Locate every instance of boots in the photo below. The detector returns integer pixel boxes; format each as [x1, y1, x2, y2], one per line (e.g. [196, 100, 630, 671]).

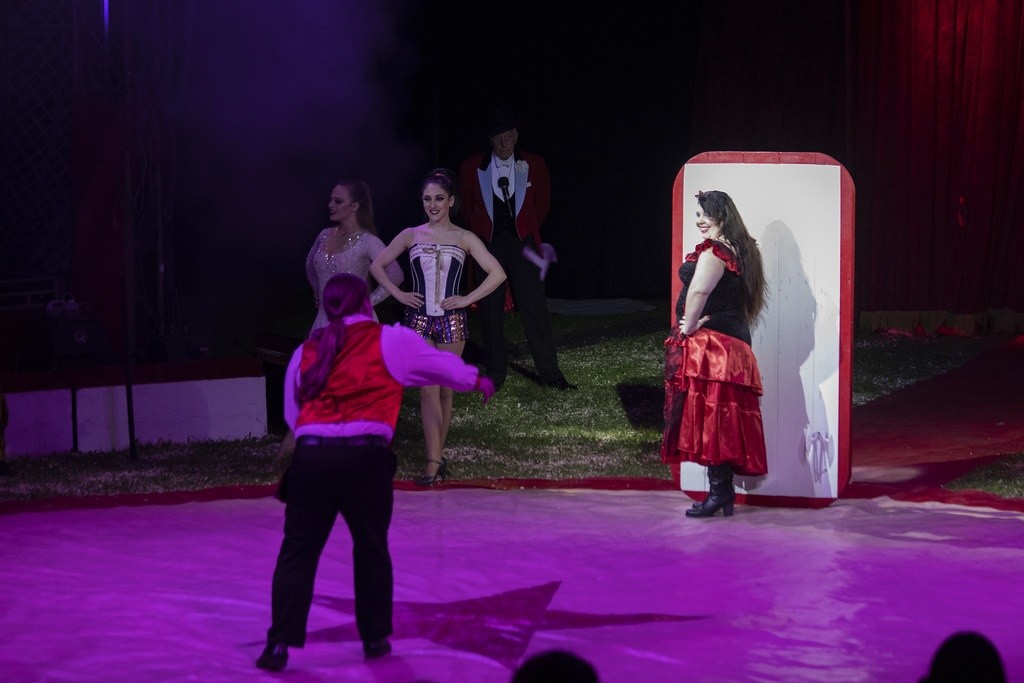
[685, 463, 734, 517]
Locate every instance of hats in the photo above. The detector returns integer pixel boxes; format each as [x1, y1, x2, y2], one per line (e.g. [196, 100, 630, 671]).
[484, 106, 520, 135]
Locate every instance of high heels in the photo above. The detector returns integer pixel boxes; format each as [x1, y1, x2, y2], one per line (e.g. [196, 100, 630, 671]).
[417, 456, 447, 485]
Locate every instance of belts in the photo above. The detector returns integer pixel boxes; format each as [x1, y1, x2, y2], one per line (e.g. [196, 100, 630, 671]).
[297, 435, 386, 447]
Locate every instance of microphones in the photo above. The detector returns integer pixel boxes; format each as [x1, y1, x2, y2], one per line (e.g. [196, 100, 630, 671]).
[497, 176, 513, 216]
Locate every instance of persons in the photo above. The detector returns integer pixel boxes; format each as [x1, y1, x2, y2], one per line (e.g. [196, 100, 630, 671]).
[255, 274, 497, 670]
[661, 191, 767, 517]
[369, 168, 508, 485]
[306, 181, 405, 337]
[457, 114, 579, 391]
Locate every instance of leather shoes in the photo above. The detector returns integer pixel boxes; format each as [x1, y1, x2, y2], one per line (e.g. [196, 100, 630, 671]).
[256, 642, 287, 671]
[364, 639, 391, 658]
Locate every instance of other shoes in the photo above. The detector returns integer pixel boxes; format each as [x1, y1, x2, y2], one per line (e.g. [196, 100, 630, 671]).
[493, 383, 502, 392]
[541, 377, 577, 391]
